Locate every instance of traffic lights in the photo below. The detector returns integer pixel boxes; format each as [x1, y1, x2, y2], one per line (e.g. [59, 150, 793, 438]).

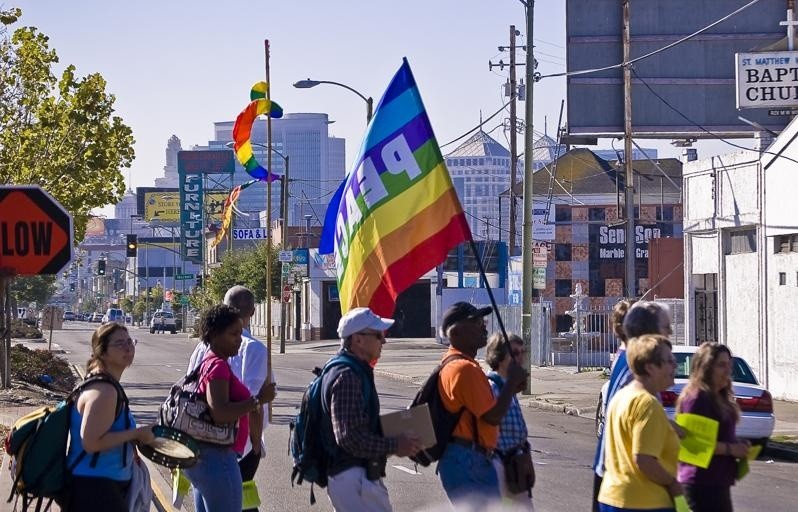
[126, 233, 137, 257]
[98, 261, 105, 275]
[70, 283, 74, 291]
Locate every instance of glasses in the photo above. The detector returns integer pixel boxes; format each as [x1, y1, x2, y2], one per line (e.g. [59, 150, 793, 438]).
[108, 339, 137, 348]
[357, 331, 382, 339]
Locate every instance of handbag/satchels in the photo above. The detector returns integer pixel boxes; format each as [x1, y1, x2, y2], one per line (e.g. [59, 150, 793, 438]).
[159, 355, 240, 446]
[500, 441, 536, 493]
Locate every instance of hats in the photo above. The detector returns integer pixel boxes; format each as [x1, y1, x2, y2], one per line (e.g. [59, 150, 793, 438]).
[442, 302, 493, 338]
[336, 307, 396, 338]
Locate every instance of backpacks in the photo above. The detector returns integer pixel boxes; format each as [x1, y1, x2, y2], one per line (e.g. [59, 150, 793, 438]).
[287, 355, 372, 485]
[407, 354, 468, 466]
[5, 374, 126, 496]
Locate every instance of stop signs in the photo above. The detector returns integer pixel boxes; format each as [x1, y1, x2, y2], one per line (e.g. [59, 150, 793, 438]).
[282, 286, 291, 303]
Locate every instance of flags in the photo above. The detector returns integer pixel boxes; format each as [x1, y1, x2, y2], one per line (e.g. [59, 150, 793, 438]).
[318, 61, 471, 371]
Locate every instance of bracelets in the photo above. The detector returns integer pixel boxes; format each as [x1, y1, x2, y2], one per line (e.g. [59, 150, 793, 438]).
[252, 395, 261, 412]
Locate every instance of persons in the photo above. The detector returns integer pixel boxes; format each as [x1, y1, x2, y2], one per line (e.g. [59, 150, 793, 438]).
[59, 321, 161, 512]
[589, 297, 750, 510]
[182, 303, 276, 511]
[322, 307, 426, 511]
[435, 301, 530, 511]
[187, 284, 271, 511]
[484, 331, 537, 511]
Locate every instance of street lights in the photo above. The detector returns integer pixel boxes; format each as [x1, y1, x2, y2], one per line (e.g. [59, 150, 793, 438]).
[160, 195, 218, 276]
[290, 77, 374, 135]
[226, 139, 288, 354]
[570, 282, 588, 372]
[142, 224, 175, 295]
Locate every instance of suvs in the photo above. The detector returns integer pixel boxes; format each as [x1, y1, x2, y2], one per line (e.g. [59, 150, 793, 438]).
[149, 311, 177, 335]
[102, 309, 124, 325]
[63, 311, 74, 322]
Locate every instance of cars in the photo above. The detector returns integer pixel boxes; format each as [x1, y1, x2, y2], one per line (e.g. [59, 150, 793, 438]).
[595, 343, 780, 457]
[75, 311, 103, 323]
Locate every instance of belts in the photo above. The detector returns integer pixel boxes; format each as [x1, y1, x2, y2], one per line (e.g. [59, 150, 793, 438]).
[450, 436, 494, 460]
